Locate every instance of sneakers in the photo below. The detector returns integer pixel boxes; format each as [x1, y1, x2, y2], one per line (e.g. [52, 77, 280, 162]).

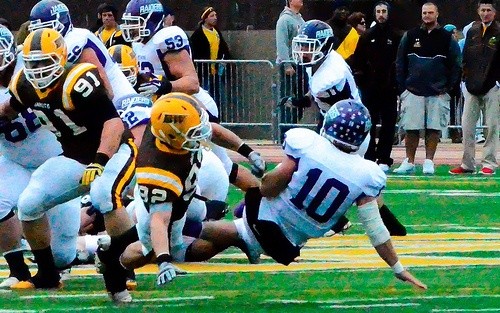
[95, 243, 108, 273]
[393, 156, 436, 174]
[475, 133, 485, 142]
[118, 253, 137, 289]
[378, 163, 389, 171]
[233, 216, 261, 264]
[234, 198, 246, 218]
[103, 261, 132, 303]
[323, 215, 351, 236]
[449, 165, 497, 174]
[11, 274, 63, 291]
[0, 272, 32, 288]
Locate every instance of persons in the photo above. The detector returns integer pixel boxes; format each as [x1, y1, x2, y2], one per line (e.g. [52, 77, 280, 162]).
[0, 0, 500, 304]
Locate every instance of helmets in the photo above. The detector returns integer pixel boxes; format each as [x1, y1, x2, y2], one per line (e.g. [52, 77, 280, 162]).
[443, 23, 457, 32]
[0, 0, 70, 73]
[297, 18, 334, 58]
[319, 98, 371, 150]
[150, 91, 208, 151]
[125, 0, 165, 36]
[113, 93, 153, 128]
[107, 44, 139, 76]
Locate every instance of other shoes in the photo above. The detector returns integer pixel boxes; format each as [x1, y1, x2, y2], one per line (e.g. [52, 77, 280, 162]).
[451, 133, 462, 143]
[440, 137, 452, 143]
[401, 138, 425, 146]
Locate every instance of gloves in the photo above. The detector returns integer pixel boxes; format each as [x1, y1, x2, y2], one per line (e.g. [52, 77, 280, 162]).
[156, 261, 187, 284]
[276, 95, 298, 108]
[79, 162, 105, 184]
[138, 71, 172, 96]
[249, 150, 266, 176]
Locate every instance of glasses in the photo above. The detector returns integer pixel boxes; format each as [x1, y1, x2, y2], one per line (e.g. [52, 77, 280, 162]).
[358, 20, 366, 25]
